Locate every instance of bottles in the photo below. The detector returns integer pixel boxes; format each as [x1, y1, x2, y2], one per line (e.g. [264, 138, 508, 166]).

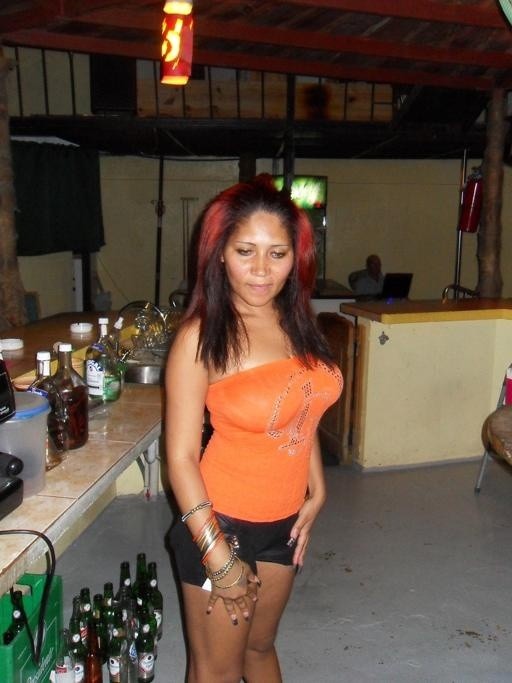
[5, 552, 165, 683]
[25, 312, 128, 471]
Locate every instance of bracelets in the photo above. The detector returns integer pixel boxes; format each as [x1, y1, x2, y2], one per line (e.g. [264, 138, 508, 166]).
[182, 501, 243, 588]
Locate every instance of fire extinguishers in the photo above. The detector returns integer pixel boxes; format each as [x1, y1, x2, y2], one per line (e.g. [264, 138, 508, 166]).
[459, 161, 484, 233]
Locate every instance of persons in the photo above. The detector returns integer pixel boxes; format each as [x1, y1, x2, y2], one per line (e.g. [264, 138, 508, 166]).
[354, 254, 385, 295]
[164, 175, 344, 683]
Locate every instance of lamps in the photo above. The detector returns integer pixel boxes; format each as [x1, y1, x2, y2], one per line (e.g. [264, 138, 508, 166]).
[158, 13, 195, 86]
[161, 0, 193, 15]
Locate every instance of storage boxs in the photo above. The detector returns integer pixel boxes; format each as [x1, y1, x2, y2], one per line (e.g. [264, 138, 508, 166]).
[1, 573, 67, 683]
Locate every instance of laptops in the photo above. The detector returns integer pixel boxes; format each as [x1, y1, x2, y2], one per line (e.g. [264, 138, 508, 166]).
[380, 274, 412, 298]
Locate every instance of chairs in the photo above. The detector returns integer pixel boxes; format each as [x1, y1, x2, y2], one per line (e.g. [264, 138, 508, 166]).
[473, 363, 512, 494]
